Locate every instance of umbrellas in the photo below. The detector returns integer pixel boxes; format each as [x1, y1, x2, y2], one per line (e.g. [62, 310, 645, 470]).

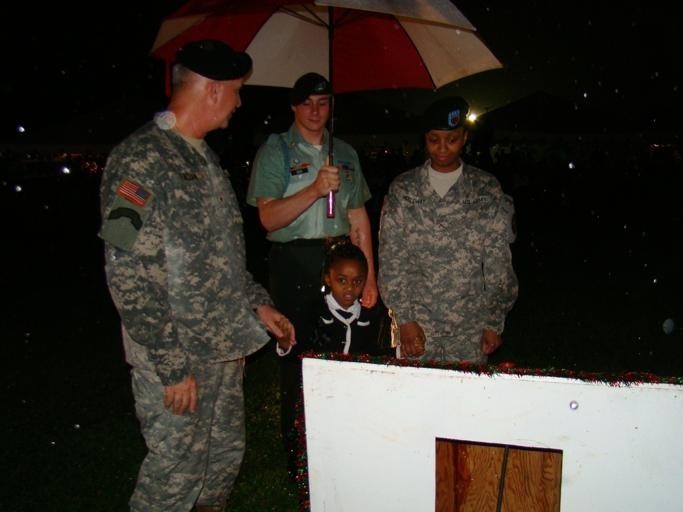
[147, 0, 505, 97]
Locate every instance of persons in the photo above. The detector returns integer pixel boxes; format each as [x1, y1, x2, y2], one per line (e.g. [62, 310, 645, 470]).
[275, 236, 426, 467]
[98, 36, 298, 511]
[374, 93, 522, 364]
[245, 71, 379, 449]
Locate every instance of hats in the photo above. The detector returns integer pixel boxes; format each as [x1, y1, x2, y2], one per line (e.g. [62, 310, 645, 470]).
[174, 39, 252, 81]
[290, 73, 333, 105]
[412, 97, 469, 134]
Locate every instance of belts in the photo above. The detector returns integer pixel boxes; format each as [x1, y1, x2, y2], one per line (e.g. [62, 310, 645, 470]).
[271, 234, 348, 249]
